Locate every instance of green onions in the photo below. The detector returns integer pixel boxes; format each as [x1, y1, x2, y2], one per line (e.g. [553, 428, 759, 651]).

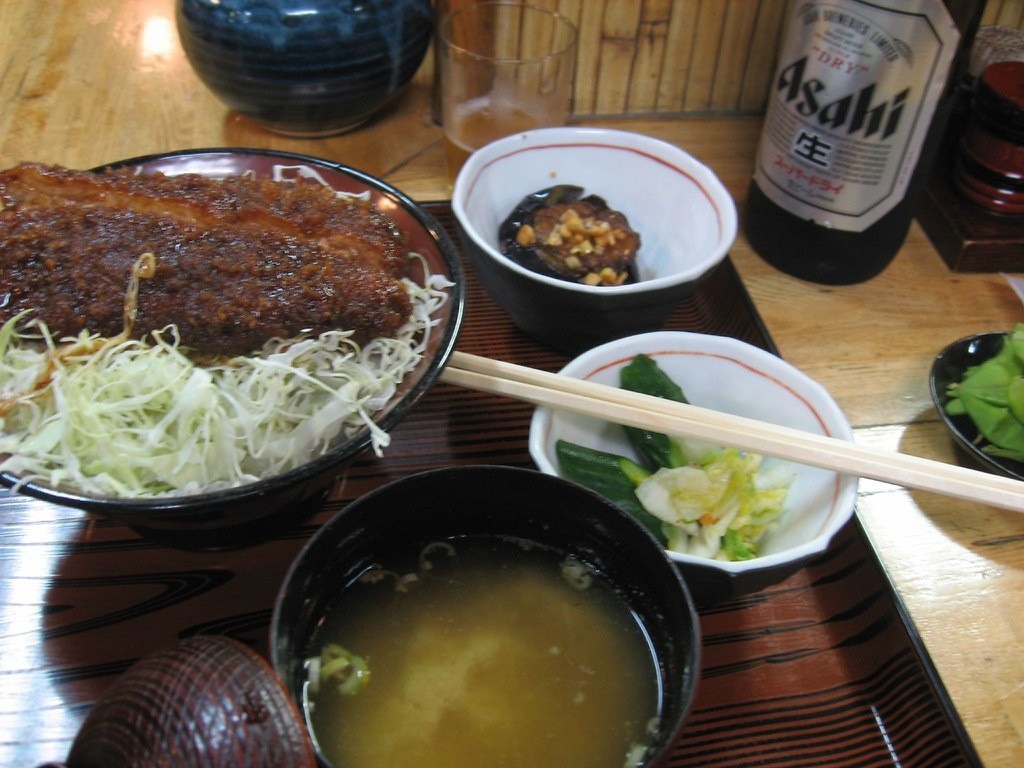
[296, 542, 663, 768]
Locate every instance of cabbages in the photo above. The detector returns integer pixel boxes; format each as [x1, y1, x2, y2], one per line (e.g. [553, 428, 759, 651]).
[635, 438, 793, 566]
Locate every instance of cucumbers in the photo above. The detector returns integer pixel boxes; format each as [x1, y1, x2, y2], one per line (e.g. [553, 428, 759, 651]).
[554, 353, 707, 545]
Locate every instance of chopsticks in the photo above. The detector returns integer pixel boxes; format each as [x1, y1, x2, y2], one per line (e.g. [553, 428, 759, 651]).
[435, 345, 1024, 528]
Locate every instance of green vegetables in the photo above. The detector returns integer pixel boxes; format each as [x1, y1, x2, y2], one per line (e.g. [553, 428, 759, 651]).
[945, 318, 1023, 469]
[0, 164, 458, 498]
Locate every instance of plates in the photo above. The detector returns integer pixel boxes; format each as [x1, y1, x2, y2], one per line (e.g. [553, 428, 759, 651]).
[430, 2, 578, 149]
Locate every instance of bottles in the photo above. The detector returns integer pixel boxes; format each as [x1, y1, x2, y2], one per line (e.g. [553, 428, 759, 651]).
[740, 0, 993, 286]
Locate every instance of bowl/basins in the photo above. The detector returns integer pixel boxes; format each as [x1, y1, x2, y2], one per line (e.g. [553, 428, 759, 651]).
[929, 329, 1024, 482]
[527, 330, 856, 598]
[0, 144, 469, 551]
[449, 127, 738, 351]
[268, 463, 700, 768]
